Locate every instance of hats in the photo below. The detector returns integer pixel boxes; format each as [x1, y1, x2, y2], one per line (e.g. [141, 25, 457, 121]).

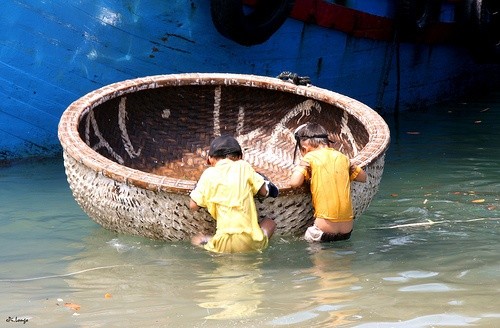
[206, 134, 242, 165]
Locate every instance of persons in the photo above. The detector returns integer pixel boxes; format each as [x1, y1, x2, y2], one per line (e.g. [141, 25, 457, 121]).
[289, 123, 367, 244]
[190, 134, 277, 257]
